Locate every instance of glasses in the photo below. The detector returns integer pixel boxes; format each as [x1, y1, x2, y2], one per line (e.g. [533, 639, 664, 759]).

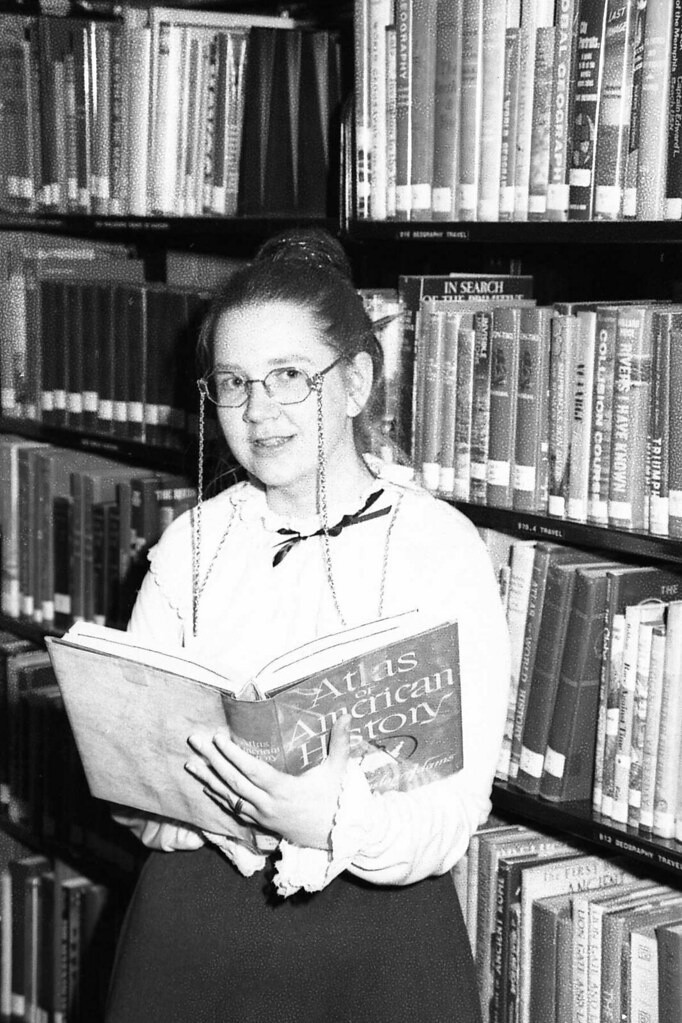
[199, 344, 367, 408]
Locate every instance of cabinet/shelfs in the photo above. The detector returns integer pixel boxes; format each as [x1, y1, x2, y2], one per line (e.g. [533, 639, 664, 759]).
[0, 0, 682, 895]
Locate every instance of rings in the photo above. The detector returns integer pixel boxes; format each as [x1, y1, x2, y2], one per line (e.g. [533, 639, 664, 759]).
[233, 798, 245, 814]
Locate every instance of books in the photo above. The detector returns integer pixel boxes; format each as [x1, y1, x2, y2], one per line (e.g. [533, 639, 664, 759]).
[2, 1, 682, 1021]
[43, 607, 474, 859]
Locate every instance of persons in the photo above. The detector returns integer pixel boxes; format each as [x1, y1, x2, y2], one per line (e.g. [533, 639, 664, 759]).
[102, 235, 514, 1023]
